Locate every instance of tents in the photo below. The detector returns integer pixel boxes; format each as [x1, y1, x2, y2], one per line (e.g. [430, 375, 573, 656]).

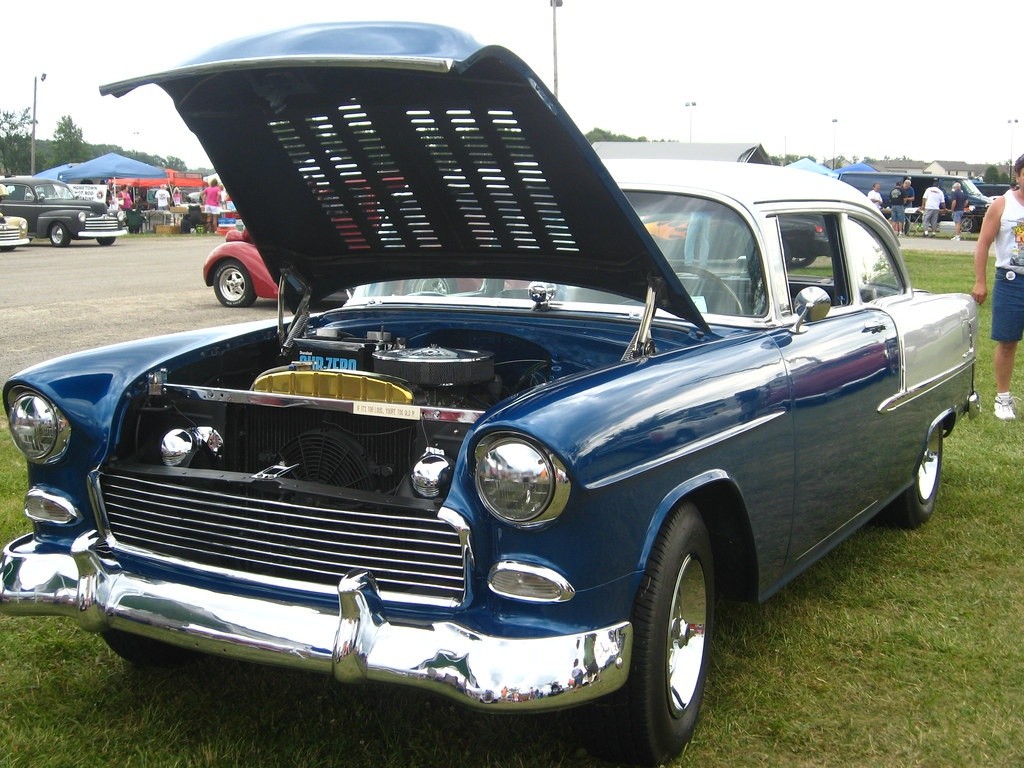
[108, 169, 209, 204]
[57, 152, 177, 207]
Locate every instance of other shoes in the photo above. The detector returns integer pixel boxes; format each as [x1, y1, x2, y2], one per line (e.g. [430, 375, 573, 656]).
[214, 230, 217, 234]
[929, 234, 936, 238]
[921, 234, 928, 237]
[207, 230, 210, 234]
[951, 236, 960, 241]
[898, 233, 905, 236]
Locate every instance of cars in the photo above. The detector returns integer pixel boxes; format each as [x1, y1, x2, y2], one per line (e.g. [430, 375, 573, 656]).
[0, 24, 979, 768]
[203, 178, 408, 307]
[0, 213, 30, 250]
[0, 175, 127, 247]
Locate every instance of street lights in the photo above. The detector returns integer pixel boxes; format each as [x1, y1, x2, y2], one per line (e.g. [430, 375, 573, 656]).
[550, 0, 563, 100]
[1008, 118, 1018, 181]
[832, 119, 837, 169]
[684, 102, 695, 143]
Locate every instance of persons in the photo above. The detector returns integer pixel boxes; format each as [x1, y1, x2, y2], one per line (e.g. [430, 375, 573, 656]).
[971, 153, 1024, 420]
[951, 182, 968, 241]
[200, 178, 232, 234]
[100, 180, 182, 225]
[868, 180, 948, 238]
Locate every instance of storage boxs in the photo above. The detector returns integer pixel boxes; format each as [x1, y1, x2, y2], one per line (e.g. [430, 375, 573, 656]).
[156, 225, 180, 234]
[220, 211, 242, 218]
[217, 228, 237, 236]
[218, 218, 236, 227]
[170, 207, 187, 213]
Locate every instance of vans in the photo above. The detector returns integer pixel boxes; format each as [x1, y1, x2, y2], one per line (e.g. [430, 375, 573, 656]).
[841, 171, 993, 233]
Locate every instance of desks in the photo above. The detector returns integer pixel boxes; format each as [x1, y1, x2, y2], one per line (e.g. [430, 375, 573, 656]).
[157, 211, 211, 226]
[880, 208, 952, 237]
[120, 210, 157, 234]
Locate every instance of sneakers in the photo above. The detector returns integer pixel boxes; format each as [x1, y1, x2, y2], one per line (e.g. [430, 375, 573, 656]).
[993, 395, 1023, 422]
